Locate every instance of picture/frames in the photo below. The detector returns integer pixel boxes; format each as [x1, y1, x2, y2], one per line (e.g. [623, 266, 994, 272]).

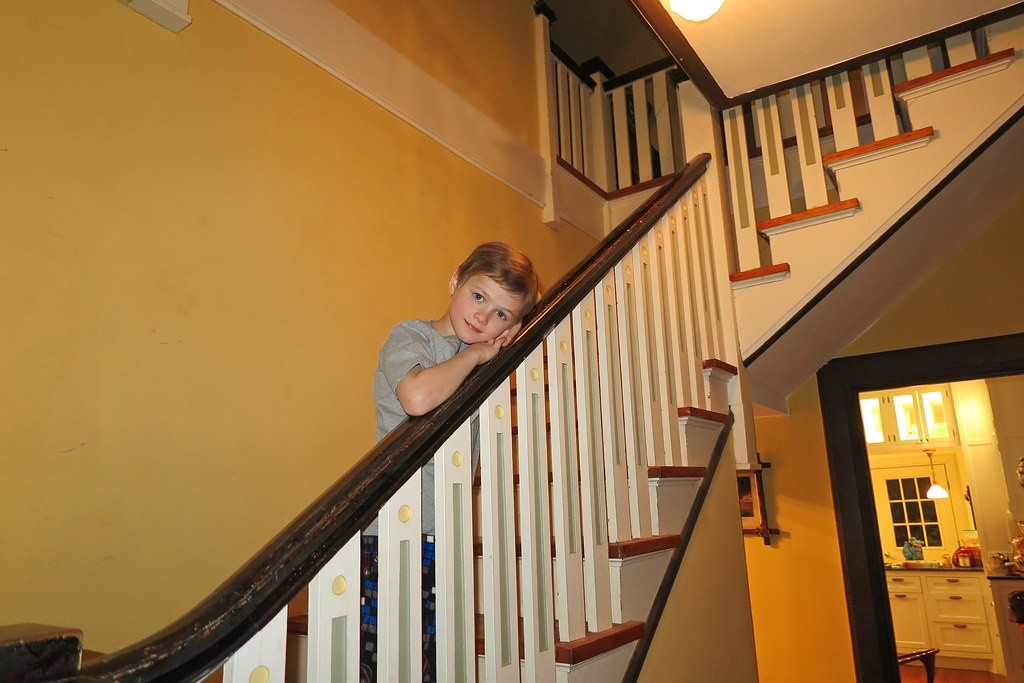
[735, 459, 778, 545]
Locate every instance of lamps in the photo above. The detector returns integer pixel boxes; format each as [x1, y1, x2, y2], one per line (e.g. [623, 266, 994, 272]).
[922, 449, 949, 499]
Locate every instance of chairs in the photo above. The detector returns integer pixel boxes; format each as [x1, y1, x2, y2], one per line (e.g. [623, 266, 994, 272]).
[897, 648, 939, 683]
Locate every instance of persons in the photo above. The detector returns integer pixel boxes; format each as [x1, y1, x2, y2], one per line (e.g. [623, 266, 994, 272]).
[360, 241, 539, 683]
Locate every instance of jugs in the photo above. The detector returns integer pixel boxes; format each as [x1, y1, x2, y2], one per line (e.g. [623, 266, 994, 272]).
[940, 553, 952, 569]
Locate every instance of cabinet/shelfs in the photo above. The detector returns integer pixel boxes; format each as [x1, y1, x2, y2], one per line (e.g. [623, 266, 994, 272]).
[858, 383, 961, 454]
[886, 571, 1004, 675]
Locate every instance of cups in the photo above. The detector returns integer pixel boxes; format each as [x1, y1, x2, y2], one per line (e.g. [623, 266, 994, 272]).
[1004, 562, 1017, 575]
